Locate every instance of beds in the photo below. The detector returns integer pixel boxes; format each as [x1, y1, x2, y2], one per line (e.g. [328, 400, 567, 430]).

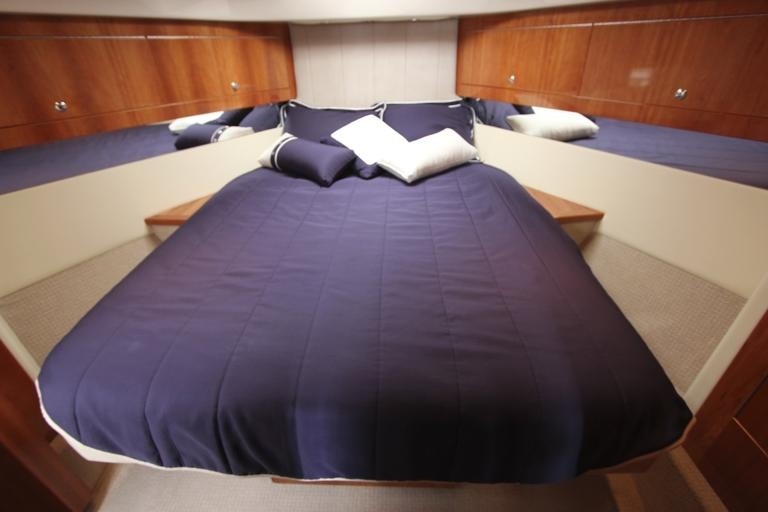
[33, 99, 702, 490]
[0, 123, 181, 198]
[564, 114, 768, 193]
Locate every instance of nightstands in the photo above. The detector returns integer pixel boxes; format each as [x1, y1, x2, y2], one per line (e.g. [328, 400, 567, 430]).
[520, 187, 605, 252]
[144, 193, 215, 243]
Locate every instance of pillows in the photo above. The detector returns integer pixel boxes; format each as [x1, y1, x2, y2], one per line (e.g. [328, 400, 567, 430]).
[168, 103, 281, 152]
[463, 96, 600, 142]
[258, 100, 485, 187]
